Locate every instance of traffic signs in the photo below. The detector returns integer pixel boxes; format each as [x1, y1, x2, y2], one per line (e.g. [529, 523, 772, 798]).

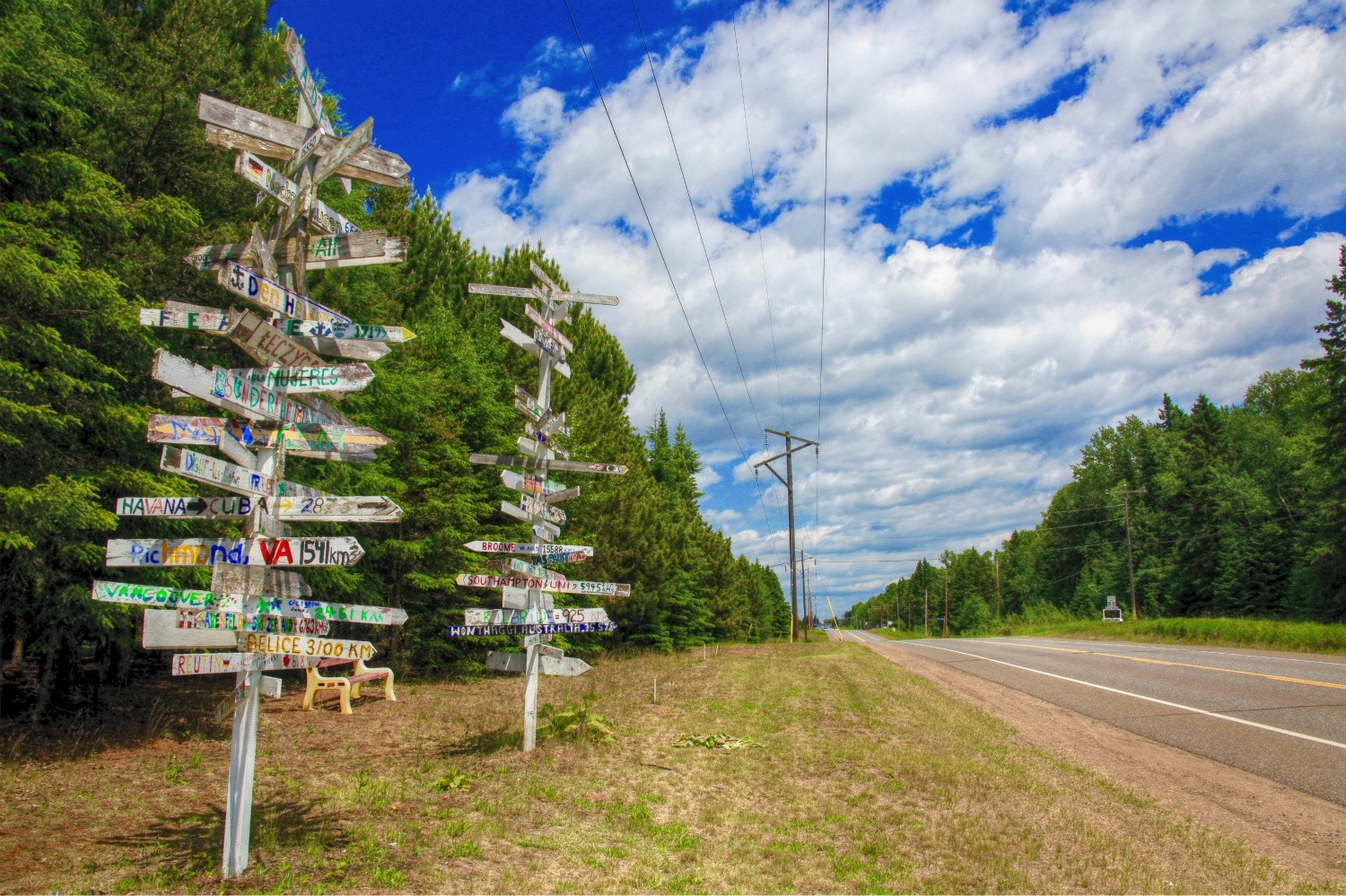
[92, 20, 421, 678]
[467, 259, 618, 380]
[449, 384, 633, 676]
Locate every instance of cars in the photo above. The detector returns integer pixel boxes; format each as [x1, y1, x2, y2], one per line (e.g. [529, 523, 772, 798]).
[823, 627, 829, 631]
[881, 624, 886, 628]
[812, 624, 819, 630]
